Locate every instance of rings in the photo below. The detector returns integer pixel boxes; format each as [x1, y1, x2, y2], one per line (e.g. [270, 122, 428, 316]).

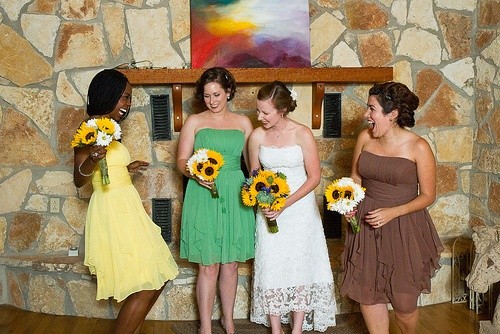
[93, 152, 99, 157]
[377, 222, 379, 225]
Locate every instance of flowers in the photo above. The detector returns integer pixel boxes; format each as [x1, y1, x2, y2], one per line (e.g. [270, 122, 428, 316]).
[325, 177, 366, 233]
[243, 168, 291, 233]
[71, 118, 121, 185]
[187, 148, 225, 200]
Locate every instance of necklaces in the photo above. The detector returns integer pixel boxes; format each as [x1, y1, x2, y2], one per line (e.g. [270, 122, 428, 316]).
[273, 120, 287, 138]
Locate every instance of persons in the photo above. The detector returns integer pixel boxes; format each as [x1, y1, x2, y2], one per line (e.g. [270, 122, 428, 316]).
[248, 81, 341, 334]
[176, 66, 254, 334]
[341, 81, 446, 334]
[73, 68, 180, 334]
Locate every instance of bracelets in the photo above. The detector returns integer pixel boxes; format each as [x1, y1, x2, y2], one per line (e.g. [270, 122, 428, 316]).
[78, 163, 95, 177]
